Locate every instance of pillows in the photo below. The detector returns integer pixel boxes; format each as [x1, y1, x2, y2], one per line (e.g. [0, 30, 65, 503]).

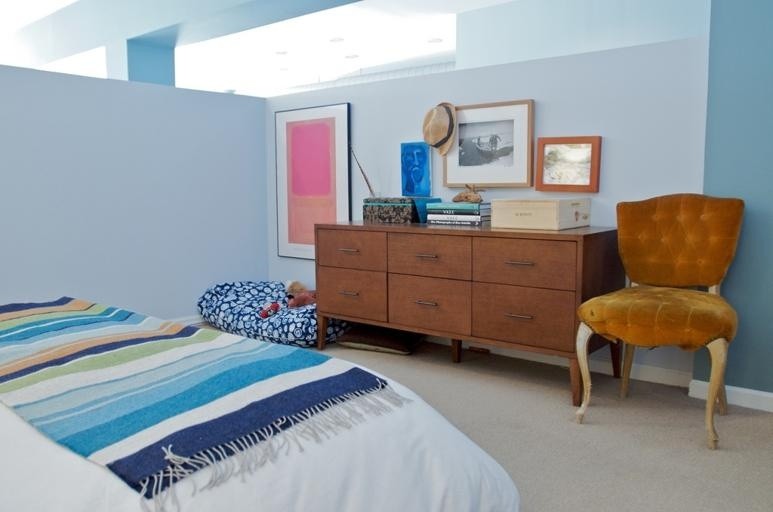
[334, 320, 427, 356]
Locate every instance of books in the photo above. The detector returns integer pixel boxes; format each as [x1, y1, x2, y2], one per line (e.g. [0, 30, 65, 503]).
[424, 200, 491, 227]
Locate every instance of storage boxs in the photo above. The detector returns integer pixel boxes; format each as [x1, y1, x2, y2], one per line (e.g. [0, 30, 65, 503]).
[360, 197, 418, 224]
[489, 199, 592, 231]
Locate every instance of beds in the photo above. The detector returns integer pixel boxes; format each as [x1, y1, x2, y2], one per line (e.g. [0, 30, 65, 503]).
[0, 296, 521, 511]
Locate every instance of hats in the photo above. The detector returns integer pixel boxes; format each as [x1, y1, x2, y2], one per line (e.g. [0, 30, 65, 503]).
[422, 103, 457, 156]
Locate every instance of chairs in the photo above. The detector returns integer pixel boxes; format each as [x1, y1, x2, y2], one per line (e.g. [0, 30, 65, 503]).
[575, 195, 747, 447]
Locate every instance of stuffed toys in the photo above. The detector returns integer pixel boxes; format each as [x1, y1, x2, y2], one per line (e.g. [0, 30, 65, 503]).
[258, 279, 317, 320]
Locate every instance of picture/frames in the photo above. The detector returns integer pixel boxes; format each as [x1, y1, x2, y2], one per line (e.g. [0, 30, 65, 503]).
[438, 103, 601, 193]
[271, 98, 355, 260]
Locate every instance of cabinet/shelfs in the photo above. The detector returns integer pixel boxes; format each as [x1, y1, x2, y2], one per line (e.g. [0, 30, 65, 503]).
[314, 219, 623, 405]
